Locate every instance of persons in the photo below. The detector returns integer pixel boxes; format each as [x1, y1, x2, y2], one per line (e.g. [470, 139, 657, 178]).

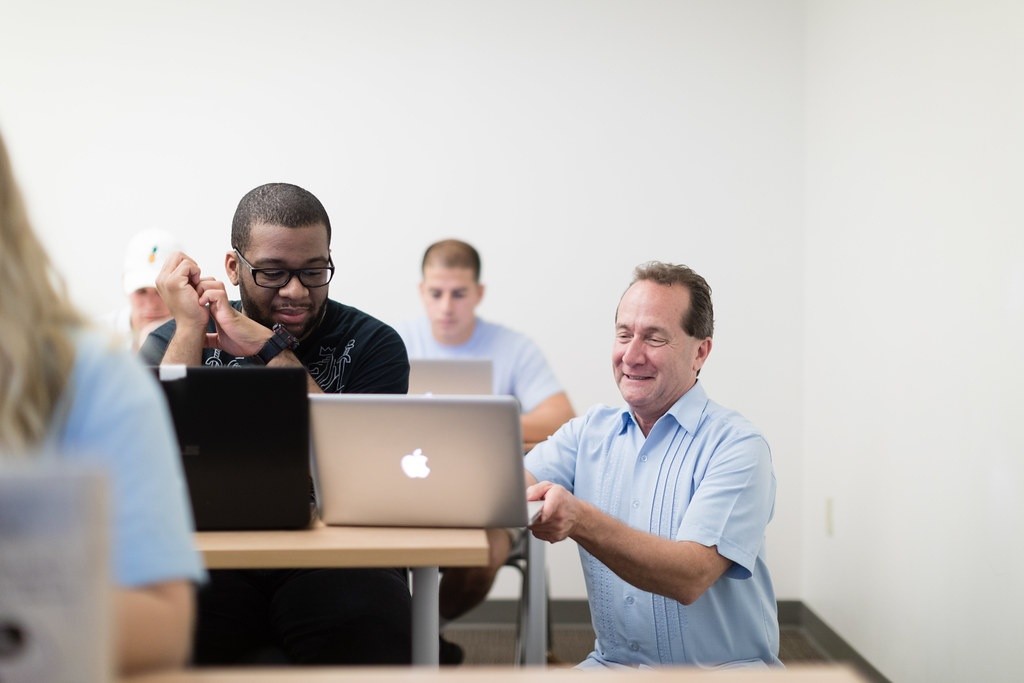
[395, 240, 577, 667]
[104, 230, 175, 353]
[135, 182, 465, 668]
[520, 257, 787, 677]
[0, 126, 209, 675]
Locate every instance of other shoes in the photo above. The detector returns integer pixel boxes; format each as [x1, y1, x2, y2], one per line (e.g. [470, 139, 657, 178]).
[439, 634, 465, 666]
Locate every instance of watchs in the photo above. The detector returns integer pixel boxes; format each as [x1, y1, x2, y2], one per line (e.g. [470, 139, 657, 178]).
[244, 322, 299, 365]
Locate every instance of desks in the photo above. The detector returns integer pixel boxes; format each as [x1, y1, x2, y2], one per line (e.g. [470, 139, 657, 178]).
[182, 502, 551, 665]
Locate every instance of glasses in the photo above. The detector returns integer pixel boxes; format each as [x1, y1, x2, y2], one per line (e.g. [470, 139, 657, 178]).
[233, 247, 335, 289]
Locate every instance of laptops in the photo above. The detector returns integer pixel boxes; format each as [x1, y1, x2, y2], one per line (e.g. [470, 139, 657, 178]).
[406, 358, 493, 396]
[308, 393, 545, 527]
[147, 365, 318, 532]
